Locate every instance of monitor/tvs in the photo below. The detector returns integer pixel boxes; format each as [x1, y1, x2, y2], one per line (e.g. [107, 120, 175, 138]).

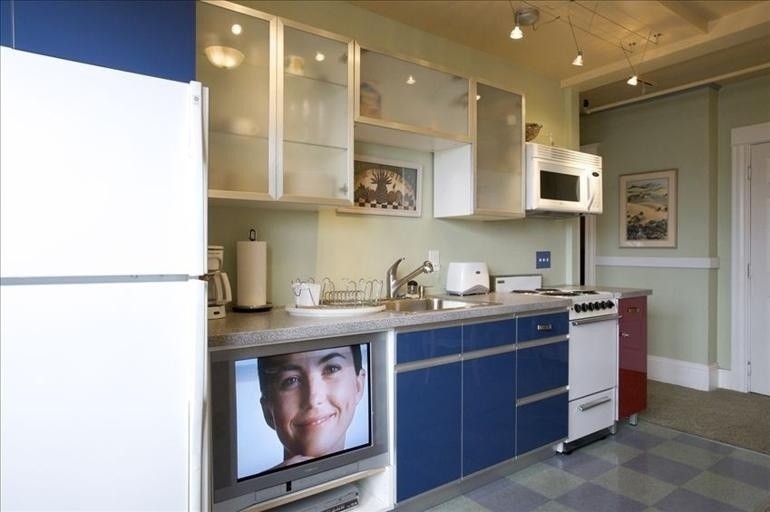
[208, 332, 392, 511]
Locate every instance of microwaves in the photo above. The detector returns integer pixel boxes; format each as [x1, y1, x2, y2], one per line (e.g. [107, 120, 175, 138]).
[526, 141, 603, 215]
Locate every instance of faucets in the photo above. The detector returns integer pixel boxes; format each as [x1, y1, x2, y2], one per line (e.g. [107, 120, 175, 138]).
[385, 257, 434, 299]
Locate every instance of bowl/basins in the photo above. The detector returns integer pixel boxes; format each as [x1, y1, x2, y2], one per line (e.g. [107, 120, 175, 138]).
[291, 282, 321, 305]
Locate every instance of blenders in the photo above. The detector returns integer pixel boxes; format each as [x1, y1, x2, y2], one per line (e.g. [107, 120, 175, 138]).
[207, 245, 233, 320]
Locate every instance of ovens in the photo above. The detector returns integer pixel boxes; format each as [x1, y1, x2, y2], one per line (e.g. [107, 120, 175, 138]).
[558, 295, 621, 455]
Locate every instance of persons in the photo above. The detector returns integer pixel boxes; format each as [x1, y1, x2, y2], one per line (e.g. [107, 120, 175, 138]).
[258, 343, 366, 472]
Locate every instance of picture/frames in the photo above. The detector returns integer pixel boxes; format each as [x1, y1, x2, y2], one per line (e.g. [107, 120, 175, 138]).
[617, 165, 680, 253]
[337, 153, 426, 219]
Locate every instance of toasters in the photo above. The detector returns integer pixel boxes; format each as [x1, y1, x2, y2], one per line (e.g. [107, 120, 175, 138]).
[445, 262, 490, 296]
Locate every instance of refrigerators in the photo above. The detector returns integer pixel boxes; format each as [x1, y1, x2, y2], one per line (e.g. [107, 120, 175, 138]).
[0, 45, 214, 512]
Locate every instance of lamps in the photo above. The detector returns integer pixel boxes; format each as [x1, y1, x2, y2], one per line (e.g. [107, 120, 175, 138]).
[313, 49, 326, 62]
[505, 2, 660, 88]
[202, 45, 245, 71]
[407, 74, 415, 84]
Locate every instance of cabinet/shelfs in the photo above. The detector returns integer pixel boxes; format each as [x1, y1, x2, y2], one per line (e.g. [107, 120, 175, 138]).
[517, 307, 569, 461]
[195, 0, 357, 208]
[395, 313, 518, 496]
[357, 42, 472, 153]
[618, 293, 652, 425]
[432, 79, 529, 223]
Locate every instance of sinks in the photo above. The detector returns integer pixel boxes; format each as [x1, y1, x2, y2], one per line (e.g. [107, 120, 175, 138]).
[373, 294, 504, 317]
